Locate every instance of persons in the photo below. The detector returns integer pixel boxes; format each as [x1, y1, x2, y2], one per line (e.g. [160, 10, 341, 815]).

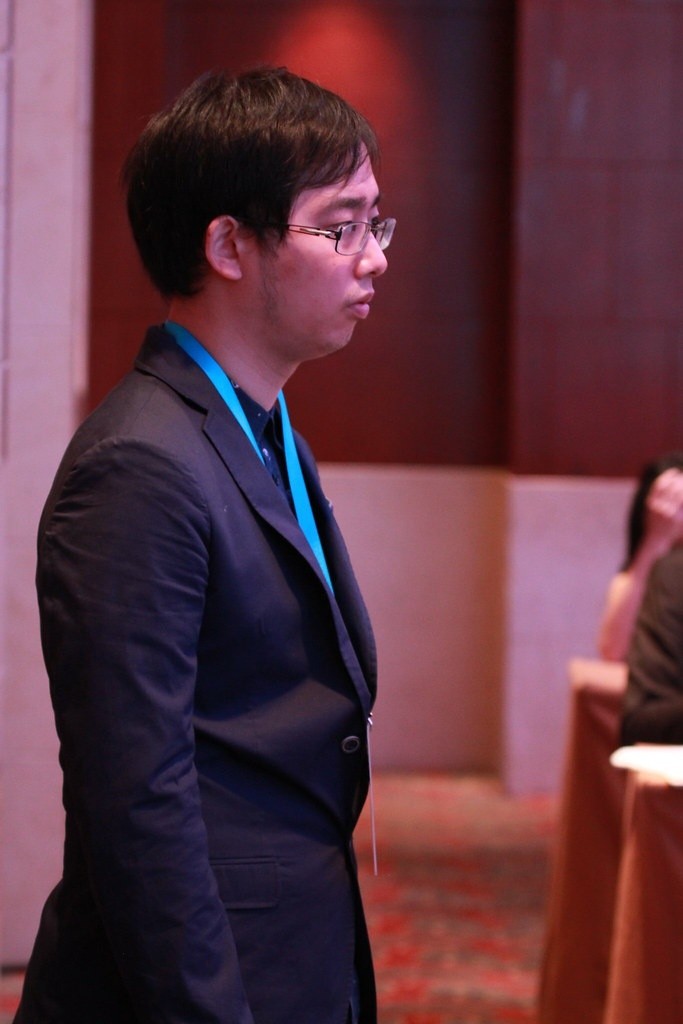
[598, 456, 683, 663]
[12, 63, 388, 1024]
[618, 538, 683, 746]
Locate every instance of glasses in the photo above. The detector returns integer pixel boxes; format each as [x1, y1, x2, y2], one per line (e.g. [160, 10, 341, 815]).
[248, 214, 398, 256]
